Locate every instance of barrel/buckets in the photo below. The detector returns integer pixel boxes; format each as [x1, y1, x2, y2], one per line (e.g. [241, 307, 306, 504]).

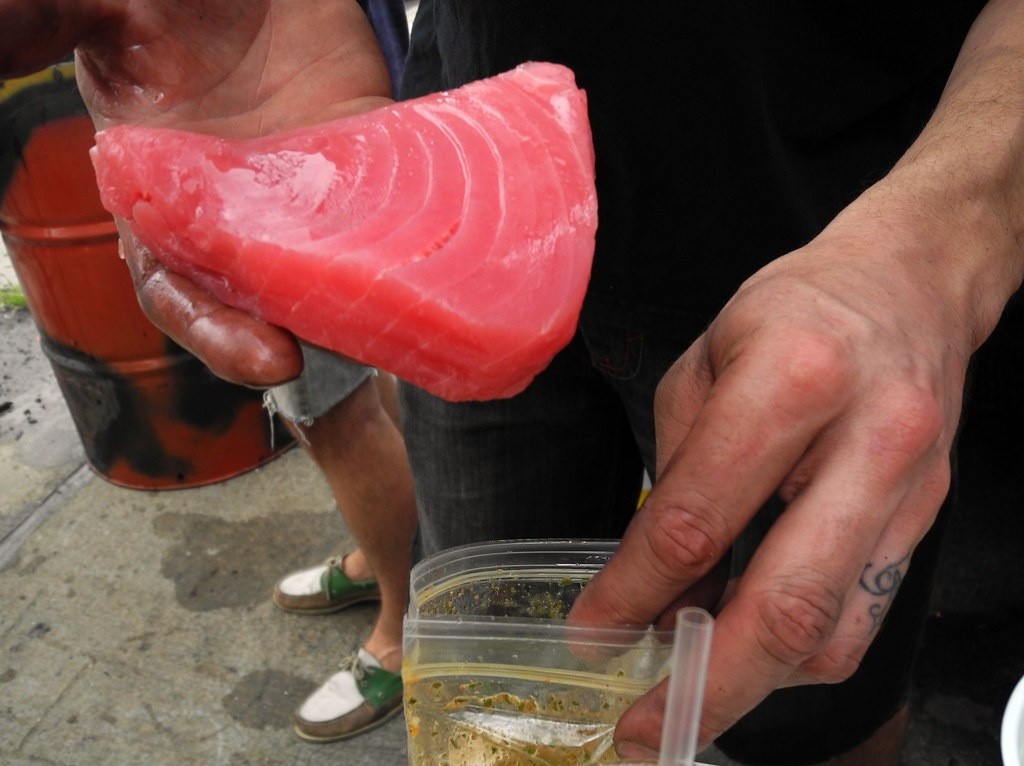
[0, 48, 299, 492]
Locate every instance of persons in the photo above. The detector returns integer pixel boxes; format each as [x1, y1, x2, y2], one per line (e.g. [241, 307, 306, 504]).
[0, 0, 1024, 766]
[262, 345, 419, 740]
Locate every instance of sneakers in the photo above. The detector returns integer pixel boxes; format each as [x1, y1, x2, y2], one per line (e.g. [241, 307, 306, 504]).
[294, 646, 403, 743]
[270, 554, 382, 614]
